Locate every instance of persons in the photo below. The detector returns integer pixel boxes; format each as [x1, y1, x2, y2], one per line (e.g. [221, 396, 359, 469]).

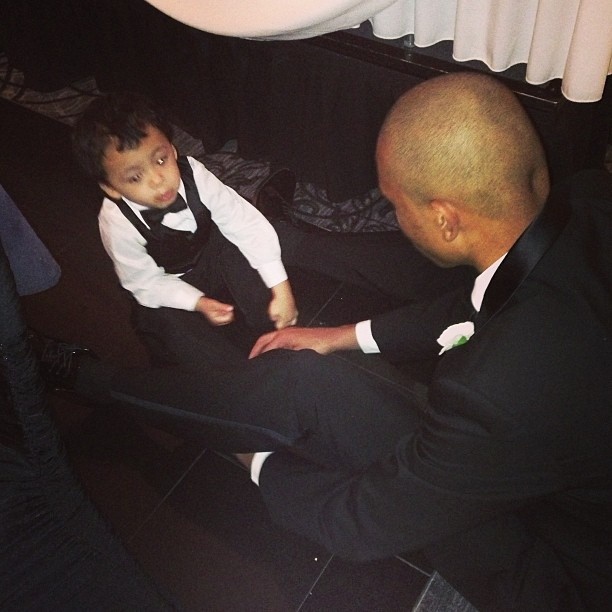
[137, 73, 612, 612]
[76, 94, 301, 349]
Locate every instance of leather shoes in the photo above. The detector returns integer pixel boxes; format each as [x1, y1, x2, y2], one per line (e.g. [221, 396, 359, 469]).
[29, 330, 116, 403]
[253, 168, 296, 224]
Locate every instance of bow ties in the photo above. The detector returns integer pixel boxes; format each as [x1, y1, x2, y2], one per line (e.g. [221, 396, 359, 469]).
[139, 192, 187, 226]
